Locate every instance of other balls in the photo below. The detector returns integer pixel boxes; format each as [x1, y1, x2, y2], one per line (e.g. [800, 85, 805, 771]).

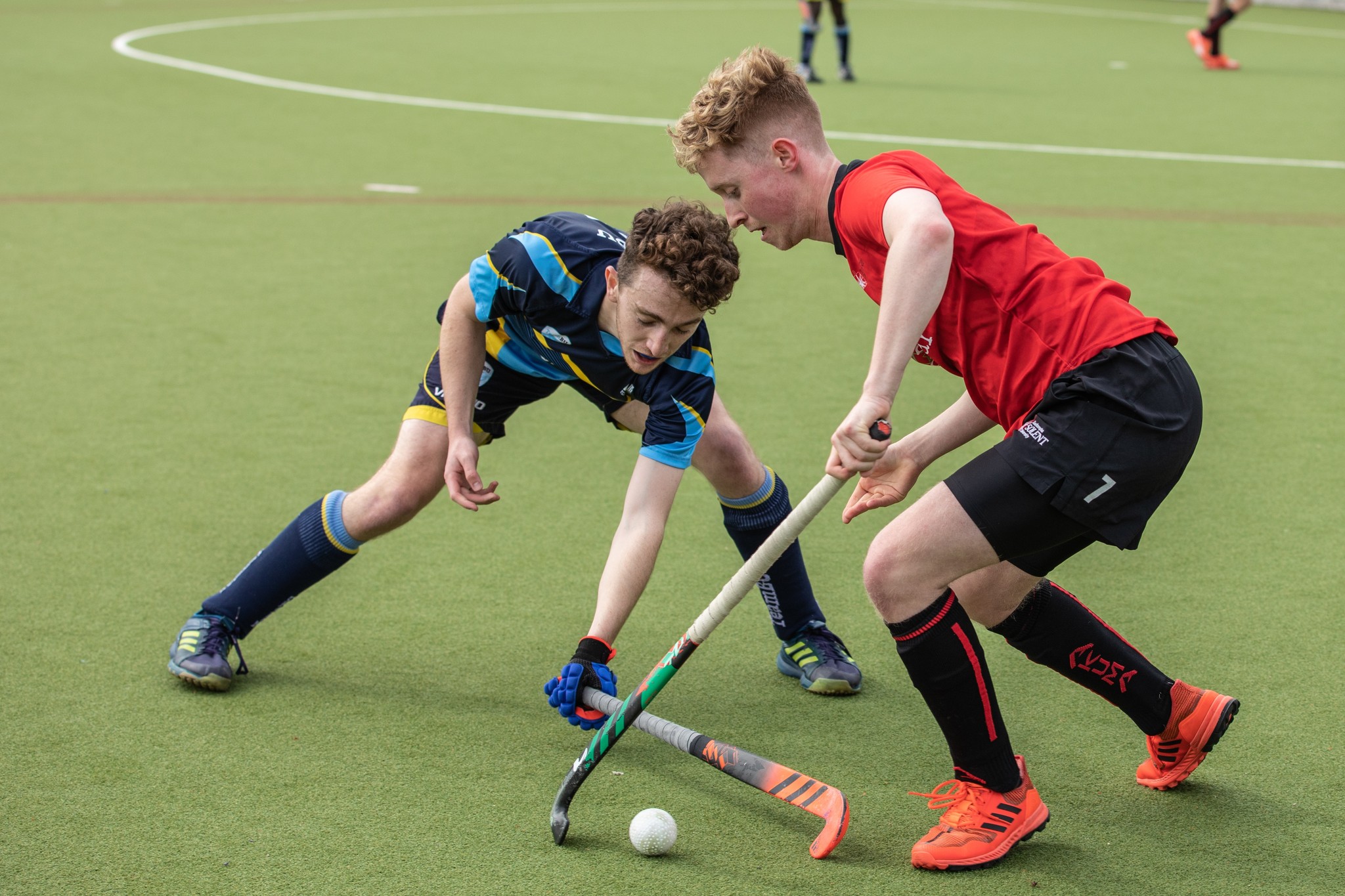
[628, 808, 677, 856]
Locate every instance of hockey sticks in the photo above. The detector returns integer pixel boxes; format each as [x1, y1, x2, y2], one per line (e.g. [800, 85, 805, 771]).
[550, 418, 894, 847]
[582, 685, 850, 858]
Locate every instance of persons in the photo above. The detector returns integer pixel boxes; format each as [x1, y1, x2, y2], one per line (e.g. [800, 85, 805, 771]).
[167, 198, 865, 695]
[665, 44, 1242, 870]
[1188, 0, 1252, 69]
[797, 0, 856, 84]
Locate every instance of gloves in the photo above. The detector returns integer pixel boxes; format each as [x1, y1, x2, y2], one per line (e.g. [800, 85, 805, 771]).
[544, 635, 618, 731]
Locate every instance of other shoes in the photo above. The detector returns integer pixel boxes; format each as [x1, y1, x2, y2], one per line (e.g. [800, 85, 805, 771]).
[838, 65, 854, 83]
[796, 63, 819, 82]
[1204, 55, 1241, 70]
[1184, 28, 1211, 66]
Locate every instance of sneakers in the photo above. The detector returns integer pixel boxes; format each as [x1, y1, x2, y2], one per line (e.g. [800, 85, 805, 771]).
[1137, 678, 1241, 790]
[776, 625, 862, 697]
[906, 757, 1052, 871]
[167, 607, 248, 692]
